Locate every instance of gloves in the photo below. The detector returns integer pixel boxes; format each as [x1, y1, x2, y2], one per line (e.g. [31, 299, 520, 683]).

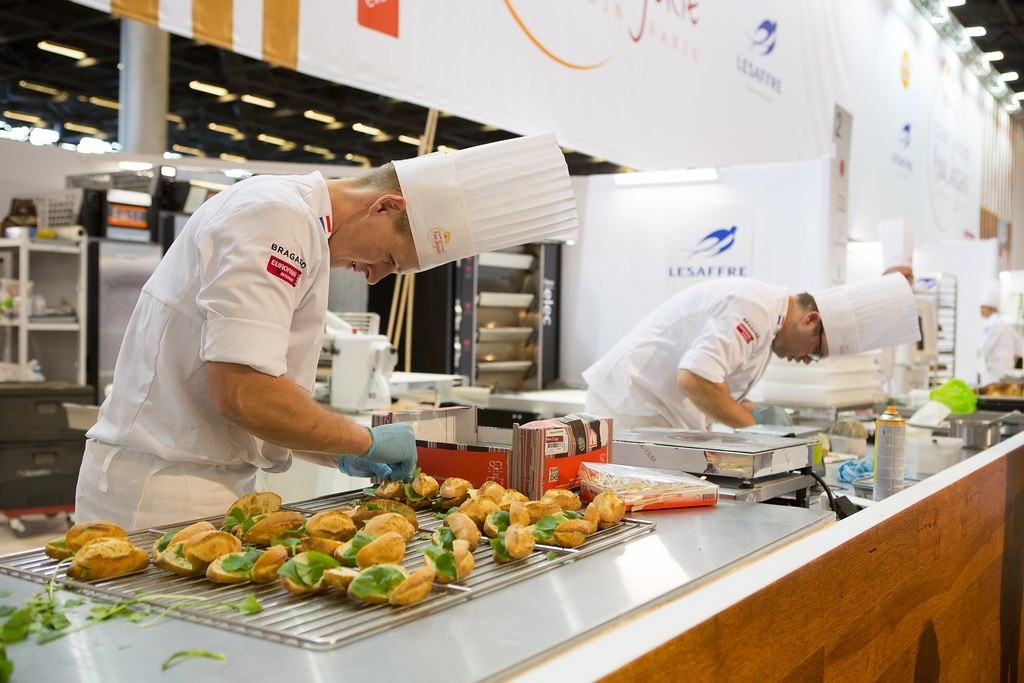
[338, 454, 392, 480]
[359, 422, 418, 481]
[752, 403, 792, 426]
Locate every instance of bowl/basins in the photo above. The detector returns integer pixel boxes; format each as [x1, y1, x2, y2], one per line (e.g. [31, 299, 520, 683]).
[6, 226, 36, 239]
[951, 421, 1001, 448]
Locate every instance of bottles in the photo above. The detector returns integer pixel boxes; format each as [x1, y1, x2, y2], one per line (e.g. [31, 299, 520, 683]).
[873, 406, 906, 502]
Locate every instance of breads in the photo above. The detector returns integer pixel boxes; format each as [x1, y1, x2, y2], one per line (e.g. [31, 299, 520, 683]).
[45, 474, 627, 607]
[971, 376, 1024, 397]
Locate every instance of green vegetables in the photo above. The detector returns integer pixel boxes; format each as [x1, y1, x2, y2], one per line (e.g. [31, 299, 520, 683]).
[0, 466, 585, 683]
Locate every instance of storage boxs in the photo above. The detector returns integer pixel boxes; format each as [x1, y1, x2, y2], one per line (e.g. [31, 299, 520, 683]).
[758, 349, 881, 409]
[579, 461, 720, 513]
[372, 403, 613, 502]
[0, 433, 90, 508]
[905, 434, 963, 477]
[1, 370, 97, 443]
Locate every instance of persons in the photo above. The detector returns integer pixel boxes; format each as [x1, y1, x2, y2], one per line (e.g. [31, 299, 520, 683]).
[75, 131, 579, 533]
[877, 217, 937, 393]
[581, 271, 921, 432]
[977, 289, 1023, 370]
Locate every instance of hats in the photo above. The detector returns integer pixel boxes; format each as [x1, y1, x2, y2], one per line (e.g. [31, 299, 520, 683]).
[980, 277, 1000, 310]
[879, 218, 914, 274]
[805, 271, 922, 359]
[391, 132, 578, 273]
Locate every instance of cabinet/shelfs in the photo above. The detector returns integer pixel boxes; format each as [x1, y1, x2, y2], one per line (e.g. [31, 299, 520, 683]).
[1, 222, 91, 384]
